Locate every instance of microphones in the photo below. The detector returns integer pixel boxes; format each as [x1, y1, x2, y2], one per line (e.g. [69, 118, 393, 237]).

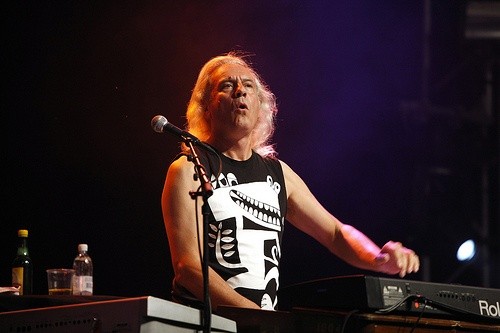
[151, 115, 215, 153]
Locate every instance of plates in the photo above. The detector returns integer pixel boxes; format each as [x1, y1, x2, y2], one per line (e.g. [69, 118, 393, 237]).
[0, 286, 20, 305]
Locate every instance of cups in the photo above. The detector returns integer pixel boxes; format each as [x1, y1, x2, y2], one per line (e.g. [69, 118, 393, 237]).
[47, 269, 73, 294]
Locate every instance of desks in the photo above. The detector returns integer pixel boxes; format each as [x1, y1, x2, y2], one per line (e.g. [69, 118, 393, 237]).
[216, 305, 500, 333]
[0, 294, 237, 333]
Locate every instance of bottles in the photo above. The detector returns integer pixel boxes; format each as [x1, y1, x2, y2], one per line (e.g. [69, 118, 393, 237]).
[11, 229, 33, 301]
[71, 242, 93, 297]
